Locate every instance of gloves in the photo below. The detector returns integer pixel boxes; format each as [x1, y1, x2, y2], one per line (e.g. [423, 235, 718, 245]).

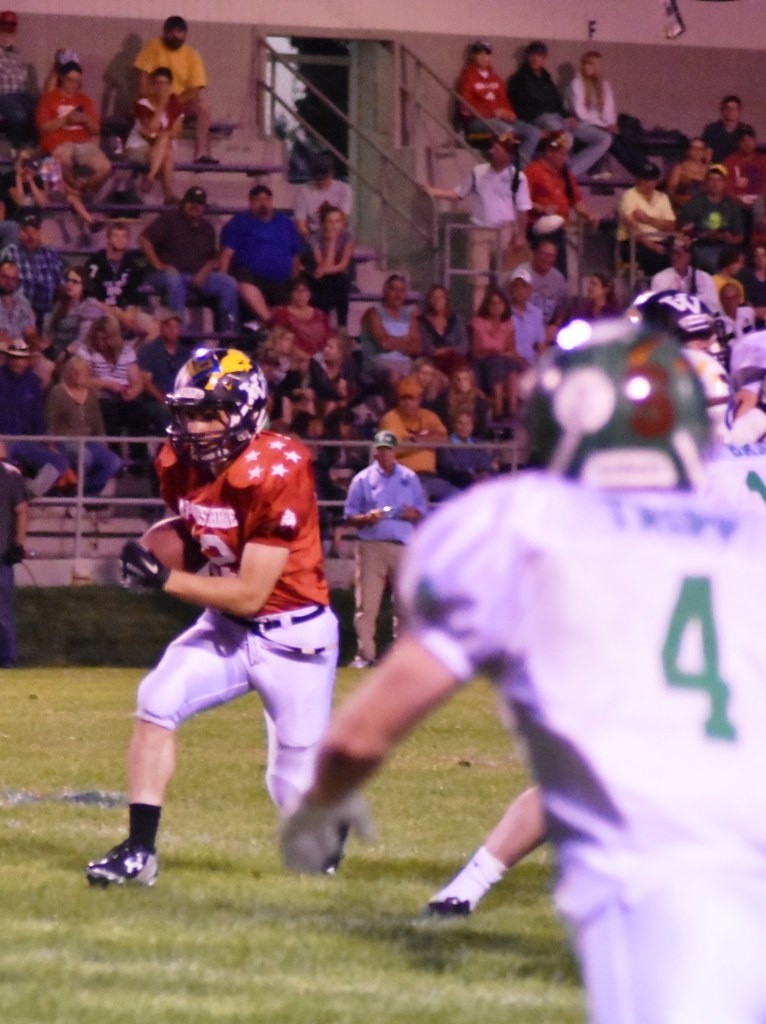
[121, 539, 172, 591]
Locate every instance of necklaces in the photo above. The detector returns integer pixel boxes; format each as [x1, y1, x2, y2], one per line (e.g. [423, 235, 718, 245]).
[400, 414, 424, 436]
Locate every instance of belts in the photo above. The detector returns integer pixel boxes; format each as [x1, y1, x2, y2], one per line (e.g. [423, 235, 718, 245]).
[221, 607, 328, 656]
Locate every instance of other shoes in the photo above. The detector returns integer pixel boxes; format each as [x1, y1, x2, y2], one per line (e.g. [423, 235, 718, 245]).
[140, 177, 153, 192]
[89, 219, 106, 233]
[163, 194, 182, 207]
[428, 897, 471, 917]
[194, 155, 220, 165]
[349, 658, 371, 669]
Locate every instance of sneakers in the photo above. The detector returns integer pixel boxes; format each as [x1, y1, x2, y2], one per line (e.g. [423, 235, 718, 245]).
[322, 821, 350, 876]
[86, 837, 159, 888]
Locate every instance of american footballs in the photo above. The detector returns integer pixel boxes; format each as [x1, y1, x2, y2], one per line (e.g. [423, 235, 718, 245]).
[139, 515, 210, 575]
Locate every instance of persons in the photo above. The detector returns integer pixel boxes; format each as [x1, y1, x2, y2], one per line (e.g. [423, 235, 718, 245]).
[0, 10, 491, 560]
[281, 322, 766, 1024]
[88, 348, 347, 889]
[344, 431, 429, 670]
[471, 40, 766, 483]
[0, 439, 29, 670]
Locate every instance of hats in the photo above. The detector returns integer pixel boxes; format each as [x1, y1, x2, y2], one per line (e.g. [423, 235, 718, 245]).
[1, 11, 16, 23]
[20, 213, 42, 228]
[396, 379, 421, 398]
[248, 184, 272, 198]
[708, 163, 725, 178]
[55, 47, 80, 64]
[312, 155, 333, 181]
[510, 269, 534, 286]
[472, 39, 491, 55]
[374, 432, 398, 450]
[184, 185, 209, 206]
[635, 162, 662, 181]
[0, 338, 34, 358]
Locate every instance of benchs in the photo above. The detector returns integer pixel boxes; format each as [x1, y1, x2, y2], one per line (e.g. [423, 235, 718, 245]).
[0, 120, 757, 586]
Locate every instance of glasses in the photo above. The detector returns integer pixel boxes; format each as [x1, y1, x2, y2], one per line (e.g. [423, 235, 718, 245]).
[60, 277, 82, 287]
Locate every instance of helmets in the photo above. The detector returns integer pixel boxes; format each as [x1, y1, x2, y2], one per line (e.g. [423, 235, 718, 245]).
[621, 289, 725, 343]
[165, 349, 271, 481]
[526, 318, 713, 499]
